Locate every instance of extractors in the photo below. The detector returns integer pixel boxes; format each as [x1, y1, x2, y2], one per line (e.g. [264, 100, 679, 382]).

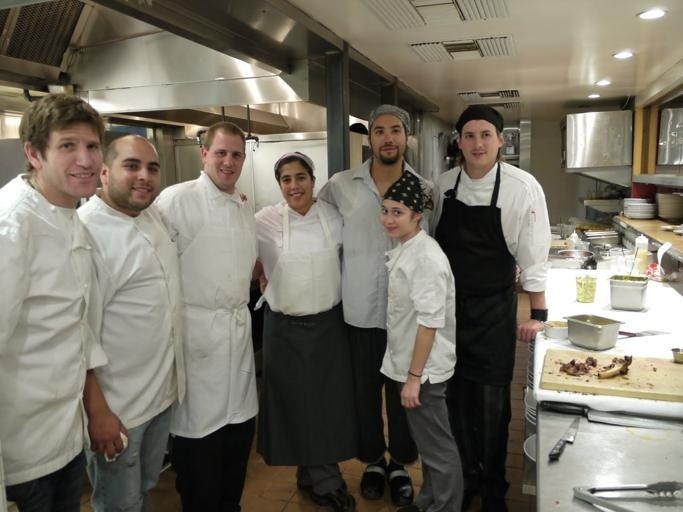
[0, 0, 292, 90]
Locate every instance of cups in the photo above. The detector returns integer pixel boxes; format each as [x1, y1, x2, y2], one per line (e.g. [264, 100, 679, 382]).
[576, 273, 596, 303]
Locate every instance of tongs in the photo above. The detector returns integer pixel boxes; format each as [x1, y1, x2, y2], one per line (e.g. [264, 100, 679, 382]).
[573, 480, 683, 512]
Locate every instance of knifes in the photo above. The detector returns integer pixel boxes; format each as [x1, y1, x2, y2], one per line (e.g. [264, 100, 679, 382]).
[549, 416, 580, 460]
[540, 401, 683, 430]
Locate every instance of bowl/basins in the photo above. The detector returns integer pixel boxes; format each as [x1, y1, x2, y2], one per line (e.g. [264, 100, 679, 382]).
[558, 250, 595, 262]
[543, 321, 568, 339]
[672, 348, 683, 364]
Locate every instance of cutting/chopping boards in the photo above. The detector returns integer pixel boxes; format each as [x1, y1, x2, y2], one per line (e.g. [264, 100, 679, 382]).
[540, 348, 683, 403]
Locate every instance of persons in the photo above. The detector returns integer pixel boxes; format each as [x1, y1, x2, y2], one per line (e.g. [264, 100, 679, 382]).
[77, 134, 186, 511]
[0, 94, 96, 511]
[162, 122, 258, 512]
[261, 106, 438, 507]
[429, 104, 551, 512]
[252, 151, 358, 509]
[379, 170, 465, 511]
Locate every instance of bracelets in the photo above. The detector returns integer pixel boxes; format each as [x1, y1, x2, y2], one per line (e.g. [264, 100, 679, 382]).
[408, 371, 422, 377]
[531, 308, 548, 321]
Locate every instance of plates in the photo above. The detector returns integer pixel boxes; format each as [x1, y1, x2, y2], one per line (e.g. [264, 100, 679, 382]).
[522, 433, 536, 463]
[656, 194, 683, 220]
[524, 390, 537, 427]
[550, 239, 568, 249]
[623, 197, 657, 219]
[527, 342, 534, 389]
[673, 230, 683, 235]
[661, 225, 680, 230]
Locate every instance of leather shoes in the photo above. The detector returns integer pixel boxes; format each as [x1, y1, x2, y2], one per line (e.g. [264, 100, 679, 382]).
[360, 457, 386, 501]
[323, 477, 355, 512]
[296, 477, 329, 505]
[386, 461, 413, 507]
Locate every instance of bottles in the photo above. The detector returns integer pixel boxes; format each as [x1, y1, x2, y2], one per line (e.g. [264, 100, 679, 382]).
[634, 234, 650, 272]
[505, 135, 516, 155]
[569, 230, 579, 250]
